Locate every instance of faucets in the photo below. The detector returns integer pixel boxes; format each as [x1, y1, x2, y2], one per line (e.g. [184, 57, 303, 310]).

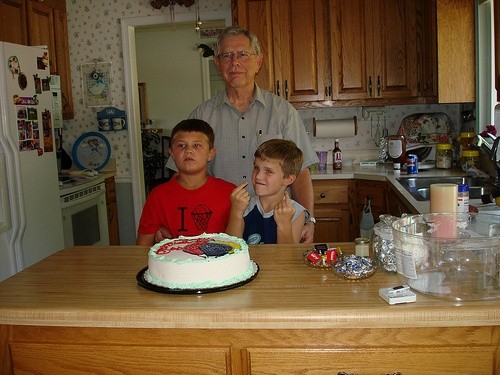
[472, 132, 498, 152]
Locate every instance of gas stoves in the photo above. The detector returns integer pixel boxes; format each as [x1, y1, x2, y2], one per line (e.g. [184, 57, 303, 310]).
[58, 170, 104, 197]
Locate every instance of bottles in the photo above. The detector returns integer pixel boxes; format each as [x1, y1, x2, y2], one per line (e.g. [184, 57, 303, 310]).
[458, 184, 469, 212]
[387, 134, 406, 171]
[372, 214, 400, 274]
[333, 139, 342, 170]
[360, 196, 374, 241]
[436, 111, 480, 172]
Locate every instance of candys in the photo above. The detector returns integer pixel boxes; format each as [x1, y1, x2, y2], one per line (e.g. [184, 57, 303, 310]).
[333, 254, 374, 276]
[374, 239, 397, 272]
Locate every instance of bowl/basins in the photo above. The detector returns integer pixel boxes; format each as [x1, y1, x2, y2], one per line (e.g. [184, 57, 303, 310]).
[391, 211, 500, 303]
[332, 258, 378, 280]
[303, 248, 343, 268]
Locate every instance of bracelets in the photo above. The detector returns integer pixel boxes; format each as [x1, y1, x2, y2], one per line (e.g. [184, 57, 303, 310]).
[307, 217, 316, 223]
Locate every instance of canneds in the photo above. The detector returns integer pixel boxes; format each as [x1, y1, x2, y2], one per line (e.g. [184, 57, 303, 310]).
[436, 131, 479, 172]
[458, 184, 469, 215]
[407, 154, 418, 174]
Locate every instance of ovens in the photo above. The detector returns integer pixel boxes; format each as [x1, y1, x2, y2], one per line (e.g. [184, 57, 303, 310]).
[60, 182, 110, 249]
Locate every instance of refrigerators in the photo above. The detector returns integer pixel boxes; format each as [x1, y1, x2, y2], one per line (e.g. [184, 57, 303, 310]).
[0, 40, 66, 283]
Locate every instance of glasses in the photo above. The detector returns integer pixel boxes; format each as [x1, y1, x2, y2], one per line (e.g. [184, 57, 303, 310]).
[218, 51, 257, 60]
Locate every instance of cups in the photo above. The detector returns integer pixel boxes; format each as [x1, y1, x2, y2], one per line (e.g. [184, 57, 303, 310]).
[97, 119, 110, 131]
[430, 183, 458, 213]
[316, 151, 328, 171]
[112, 118, 125, 130]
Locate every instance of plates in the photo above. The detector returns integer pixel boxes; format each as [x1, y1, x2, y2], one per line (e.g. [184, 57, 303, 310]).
[72, 132, 111, 171]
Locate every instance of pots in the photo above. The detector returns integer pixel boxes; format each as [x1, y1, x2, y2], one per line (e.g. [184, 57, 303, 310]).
[57, 128, 72, 170]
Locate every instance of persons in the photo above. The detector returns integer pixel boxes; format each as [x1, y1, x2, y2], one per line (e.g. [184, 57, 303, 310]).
[155, 26, 316, 244]
[136, 119, 236, 246]
[226, 139, 311, 244]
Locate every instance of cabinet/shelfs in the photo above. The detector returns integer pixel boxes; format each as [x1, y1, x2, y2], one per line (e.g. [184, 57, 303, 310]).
[311, 177, 417, 245]
[0, 0, 74, 119]
[230, 0, 477, 109]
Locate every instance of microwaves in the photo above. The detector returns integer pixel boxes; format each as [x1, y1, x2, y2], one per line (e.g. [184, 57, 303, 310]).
[50, 74, 64, 129]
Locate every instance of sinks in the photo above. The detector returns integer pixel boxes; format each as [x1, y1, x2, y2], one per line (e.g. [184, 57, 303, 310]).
[407, 188, 500, 201]
[394, 176, 488, 187]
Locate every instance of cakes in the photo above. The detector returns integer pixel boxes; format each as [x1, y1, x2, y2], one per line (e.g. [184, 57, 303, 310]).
[143, 232, 254, 289]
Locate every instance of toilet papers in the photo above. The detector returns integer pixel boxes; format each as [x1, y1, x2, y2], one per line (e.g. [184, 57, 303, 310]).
[315, 118, 355, 138]
